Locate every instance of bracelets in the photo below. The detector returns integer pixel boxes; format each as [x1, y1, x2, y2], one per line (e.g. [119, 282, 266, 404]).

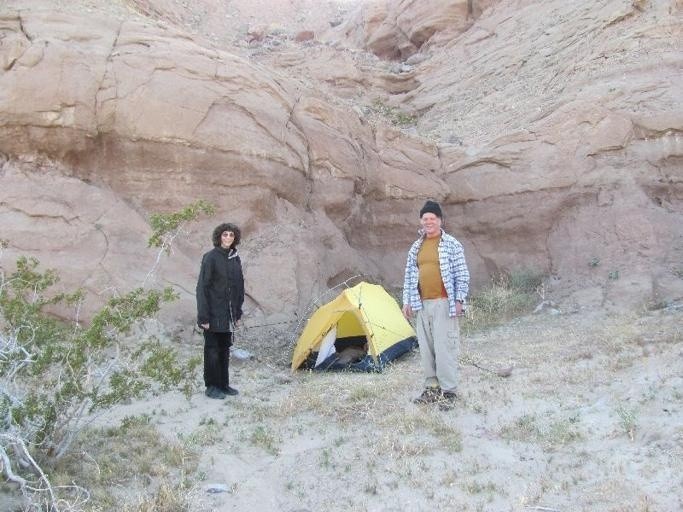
[456, 300, 463, 304]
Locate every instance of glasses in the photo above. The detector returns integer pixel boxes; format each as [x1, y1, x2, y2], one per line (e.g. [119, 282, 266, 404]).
[222, 234, 235, 238]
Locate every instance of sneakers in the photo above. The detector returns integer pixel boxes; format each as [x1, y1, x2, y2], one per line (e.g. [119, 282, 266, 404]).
[439, 393, 456, 411]
[221, 385, 238, 395]
[414, 388, 442, 404]
[206, 386, 225, 399]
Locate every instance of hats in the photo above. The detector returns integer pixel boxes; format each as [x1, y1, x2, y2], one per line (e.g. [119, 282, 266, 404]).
[421, 201, 441, 217]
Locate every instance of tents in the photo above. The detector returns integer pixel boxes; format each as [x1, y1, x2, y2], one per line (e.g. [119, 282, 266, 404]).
[283, 280, 420, 377]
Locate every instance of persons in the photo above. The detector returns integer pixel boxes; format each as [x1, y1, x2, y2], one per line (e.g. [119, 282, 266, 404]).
[193, 222, 245, 400]
[399, 199, 471, 412]
